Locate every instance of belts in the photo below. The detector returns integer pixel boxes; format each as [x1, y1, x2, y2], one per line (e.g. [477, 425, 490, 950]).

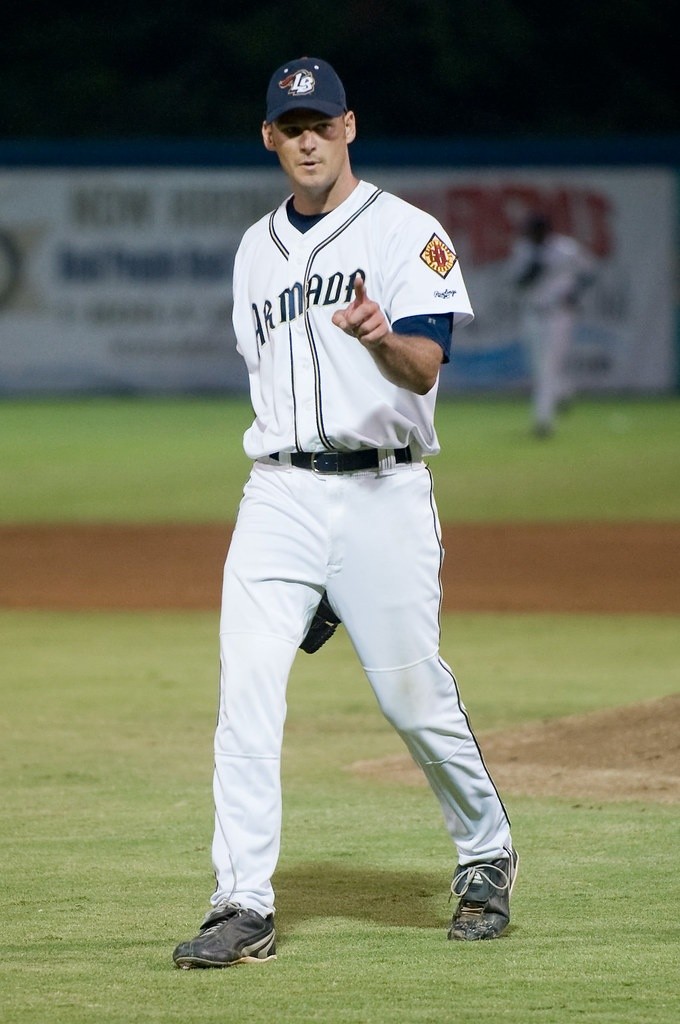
[269, 445, 413, 474]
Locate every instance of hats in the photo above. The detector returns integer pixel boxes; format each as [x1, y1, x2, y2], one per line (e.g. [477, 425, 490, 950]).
[266, 55, 349, 126]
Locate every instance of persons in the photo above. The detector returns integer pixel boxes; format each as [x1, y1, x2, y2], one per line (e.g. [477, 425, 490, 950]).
[507, 208, 611, 438]
[171, 56, 520, 970]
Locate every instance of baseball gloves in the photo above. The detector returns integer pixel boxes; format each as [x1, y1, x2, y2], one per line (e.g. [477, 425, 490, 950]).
[299, 590, 343, 655]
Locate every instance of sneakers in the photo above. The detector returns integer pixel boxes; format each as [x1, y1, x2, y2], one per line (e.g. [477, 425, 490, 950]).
[447, 846, 519, 941]
[173, 899, 277, 970]
